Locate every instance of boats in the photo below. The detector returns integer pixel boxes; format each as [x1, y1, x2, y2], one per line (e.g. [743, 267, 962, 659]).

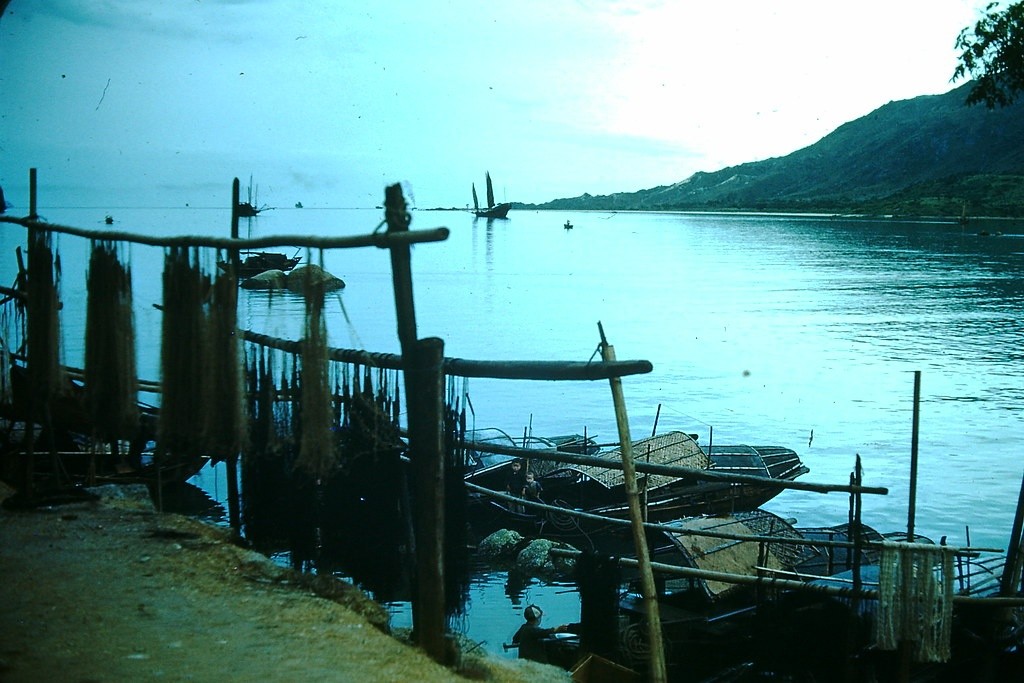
[401, 426, 1023, 683]
[216, 252, 302, 277]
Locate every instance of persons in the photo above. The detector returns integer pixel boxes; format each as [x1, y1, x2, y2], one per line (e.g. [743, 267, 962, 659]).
[506, 459, 543, 514]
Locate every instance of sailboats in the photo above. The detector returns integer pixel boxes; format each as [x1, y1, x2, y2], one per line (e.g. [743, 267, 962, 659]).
[471, 170, 512, 219]
[239, 175, 260, 217]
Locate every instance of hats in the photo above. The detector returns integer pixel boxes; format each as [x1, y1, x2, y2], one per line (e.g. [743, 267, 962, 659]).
[524, 603, 542, 620]
